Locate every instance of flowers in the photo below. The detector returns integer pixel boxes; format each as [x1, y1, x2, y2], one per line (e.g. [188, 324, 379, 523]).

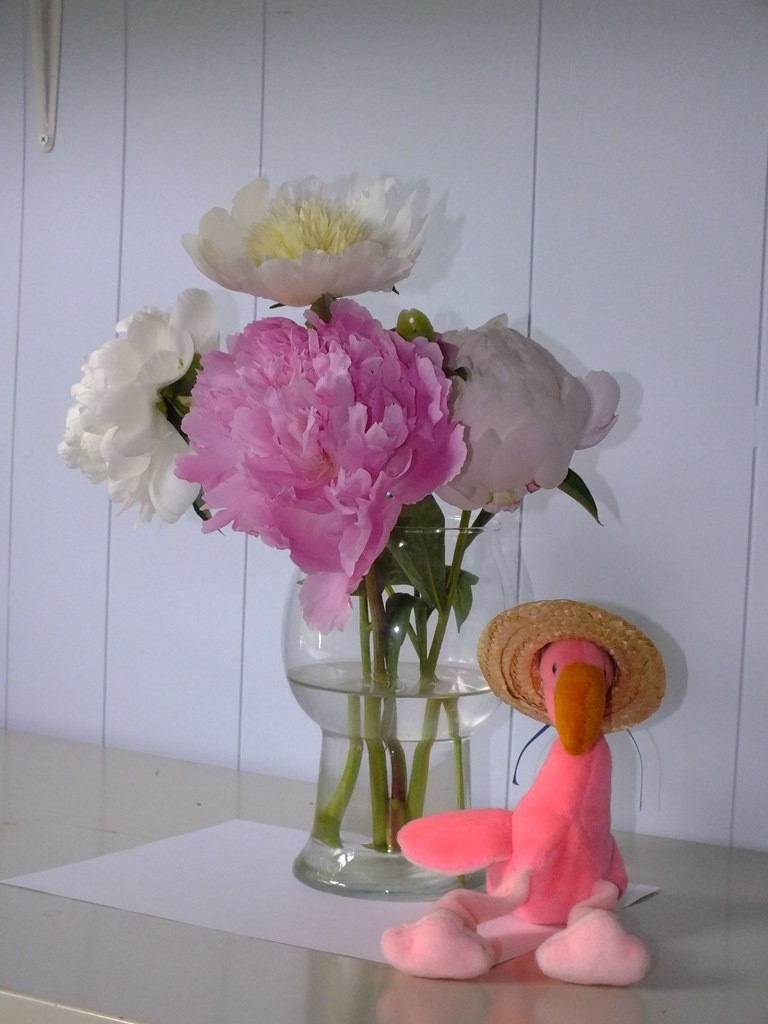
[58, 165, 622, 851]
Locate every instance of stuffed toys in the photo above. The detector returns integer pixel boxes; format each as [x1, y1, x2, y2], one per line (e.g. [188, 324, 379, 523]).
[379, 599, 667, 986]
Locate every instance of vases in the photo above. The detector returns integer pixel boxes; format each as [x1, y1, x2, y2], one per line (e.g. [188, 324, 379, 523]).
[281, 509, 524, 905]
